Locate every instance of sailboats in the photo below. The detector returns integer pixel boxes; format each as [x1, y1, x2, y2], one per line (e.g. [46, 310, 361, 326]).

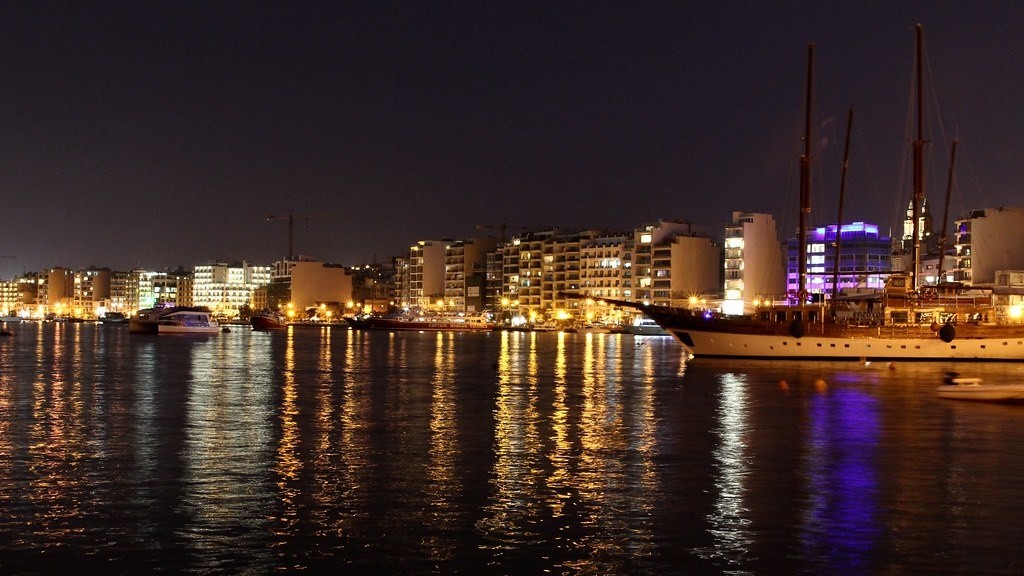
[560, 21, 1024, 362]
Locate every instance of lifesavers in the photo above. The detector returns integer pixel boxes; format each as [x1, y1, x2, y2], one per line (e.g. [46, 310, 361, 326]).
[787, 290, 796, 300]
[924, 291, 934, 300]
[798, 289, 808, 301]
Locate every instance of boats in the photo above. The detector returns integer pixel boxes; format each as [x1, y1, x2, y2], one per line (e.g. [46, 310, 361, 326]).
[936, 378, 1024, 400]
[250, 308, 288, 330]
[341, 306, 509, 332]
[607, 317, 669, 335]
[98, 306, 219, 336]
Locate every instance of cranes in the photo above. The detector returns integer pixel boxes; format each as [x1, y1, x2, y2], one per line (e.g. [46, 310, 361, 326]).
[473, 216, 543, 239]
[266, 209, 350, 259]
